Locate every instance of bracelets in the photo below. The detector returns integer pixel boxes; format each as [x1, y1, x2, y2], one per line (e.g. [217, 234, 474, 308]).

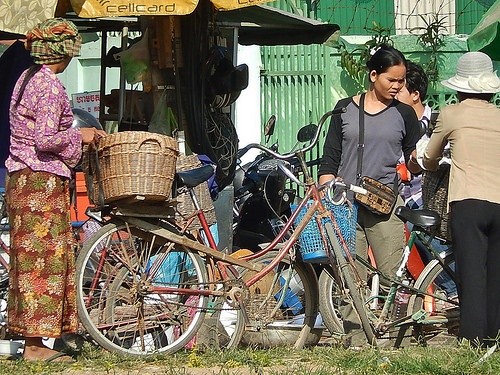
[409, 155, 417, 164]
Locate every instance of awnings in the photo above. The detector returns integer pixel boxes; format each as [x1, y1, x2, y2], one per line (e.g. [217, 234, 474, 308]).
[238, 6, 341, 50]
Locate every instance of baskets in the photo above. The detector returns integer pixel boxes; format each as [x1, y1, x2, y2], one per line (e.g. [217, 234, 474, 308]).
[245, 294, 284, 321]
[175, 153, 217, 230]
[80, 131, 180, 204]
[421, 159, 455, 242]
[290, 178, 358, 263]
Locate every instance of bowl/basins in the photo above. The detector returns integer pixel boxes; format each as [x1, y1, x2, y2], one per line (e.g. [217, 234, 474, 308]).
[0, 340, 19, 356]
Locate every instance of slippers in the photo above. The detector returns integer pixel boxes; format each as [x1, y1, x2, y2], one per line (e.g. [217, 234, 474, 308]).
[19, 345, 81, 364]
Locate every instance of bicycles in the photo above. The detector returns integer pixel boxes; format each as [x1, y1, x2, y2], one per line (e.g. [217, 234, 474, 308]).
[0, 190, 246, 354]
[207, 160, 464, 354]
[317, 243, 380, 340]
[74, 108, 384, 353]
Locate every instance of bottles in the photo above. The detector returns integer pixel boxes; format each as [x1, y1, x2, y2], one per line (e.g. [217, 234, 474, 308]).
[390, 281, 409, 322]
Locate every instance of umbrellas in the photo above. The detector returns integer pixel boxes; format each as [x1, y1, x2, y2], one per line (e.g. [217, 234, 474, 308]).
[54, 0, 278, 133]
[467, 0, 500, 62]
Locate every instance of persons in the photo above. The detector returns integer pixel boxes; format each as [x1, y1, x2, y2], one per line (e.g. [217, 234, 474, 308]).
[422, 52, 500, 350]
[4, 17, 107, 364]
[395, 59, 461, 310]
[317, 46, 423, 353]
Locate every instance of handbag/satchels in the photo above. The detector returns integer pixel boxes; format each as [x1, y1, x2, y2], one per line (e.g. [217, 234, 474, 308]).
[354, 176, 397, 216]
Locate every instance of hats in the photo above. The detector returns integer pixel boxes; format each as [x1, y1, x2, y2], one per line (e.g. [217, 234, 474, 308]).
[441, 52, 500, 93]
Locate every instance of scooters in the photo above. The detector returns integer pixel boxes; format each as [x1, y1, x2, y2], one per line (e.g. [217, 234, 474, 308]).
[217, 112, 324, 267]
